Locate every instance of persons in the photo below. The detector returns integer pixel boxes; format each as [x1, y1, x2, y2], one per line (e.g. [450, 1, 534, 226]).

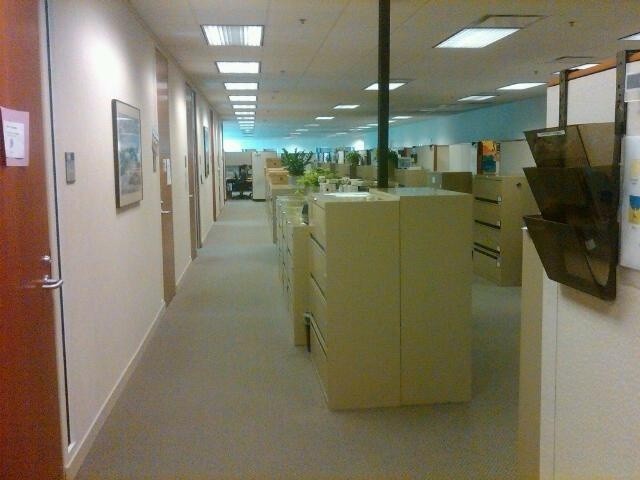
[239, 165, 248, 198]
[247, 165, 253, 175]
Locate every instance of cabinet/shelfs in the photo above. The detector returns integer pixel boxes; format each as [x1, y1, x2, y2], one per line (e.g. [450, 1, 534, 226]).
[371, 186, 473, 407]
[427, 171, 472, 193]
[222, 139, 310, 350]
[394, 169, 427, 184]
[308, 189, 402, 411]
[470, 173, 539, 292]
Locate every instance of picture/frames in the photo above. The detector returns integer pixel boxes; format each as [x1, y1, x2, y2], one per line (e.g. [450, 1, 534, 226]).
[111, 99, 144, 209]
